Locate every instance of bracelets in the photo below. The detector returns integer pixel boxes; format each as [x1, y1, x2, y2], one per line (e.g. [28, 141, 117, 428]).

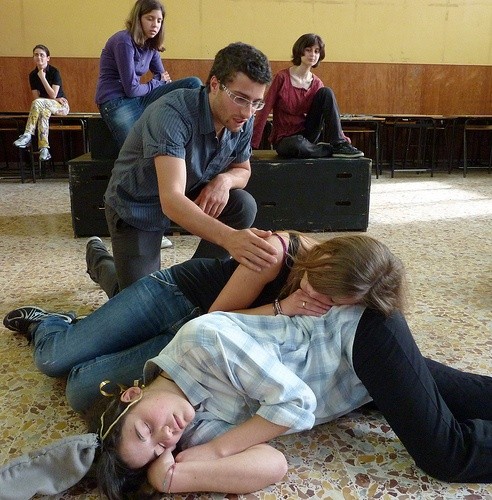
[162, 462, 176, 493]
[272, 299, 284, 316]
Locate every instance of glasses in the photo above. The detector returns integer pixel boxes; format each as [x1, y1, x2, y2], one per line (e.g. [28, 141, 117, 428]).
[218, 79, 266, 111]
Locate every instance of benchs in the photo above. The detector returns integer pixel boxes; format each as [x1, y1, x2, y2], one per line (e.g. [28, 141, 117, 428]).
[68, 150, 372, 237]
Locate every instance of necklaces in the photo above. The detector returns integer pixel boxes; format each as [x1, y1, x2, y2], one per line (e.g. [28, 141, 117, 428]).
[159, 373, 175, 383]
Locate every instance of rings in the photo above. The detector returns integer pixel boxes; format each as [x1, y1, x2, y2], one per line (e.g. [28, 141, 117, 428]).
[302, 301, 306, 307]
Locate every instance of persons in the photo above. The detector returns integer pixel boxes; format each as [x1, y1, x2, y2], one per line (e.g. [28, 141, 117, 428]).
[89, 303, 492, 495]
[94, 0, 202, 154]
[13, 44, 70, 161]
[86, 40, 278, 300]
[251, 33, 364, 159]
[2, 229, 404, 417]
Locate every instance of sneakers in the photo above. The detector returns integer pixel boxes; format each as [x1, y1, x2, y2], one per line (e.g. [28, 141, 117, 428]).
[12, 134, 33, 149]
[39, 148, 52, 162]
[3, 306, 88, 340]
[317, 142, 365, 158]
[161, 234, 172, 249]
[85, 236, 105, 283]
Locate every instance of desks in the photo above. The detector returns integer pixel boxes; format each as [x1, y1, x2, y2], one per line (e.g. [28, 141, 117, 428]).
[0, 111, 492, 184]
